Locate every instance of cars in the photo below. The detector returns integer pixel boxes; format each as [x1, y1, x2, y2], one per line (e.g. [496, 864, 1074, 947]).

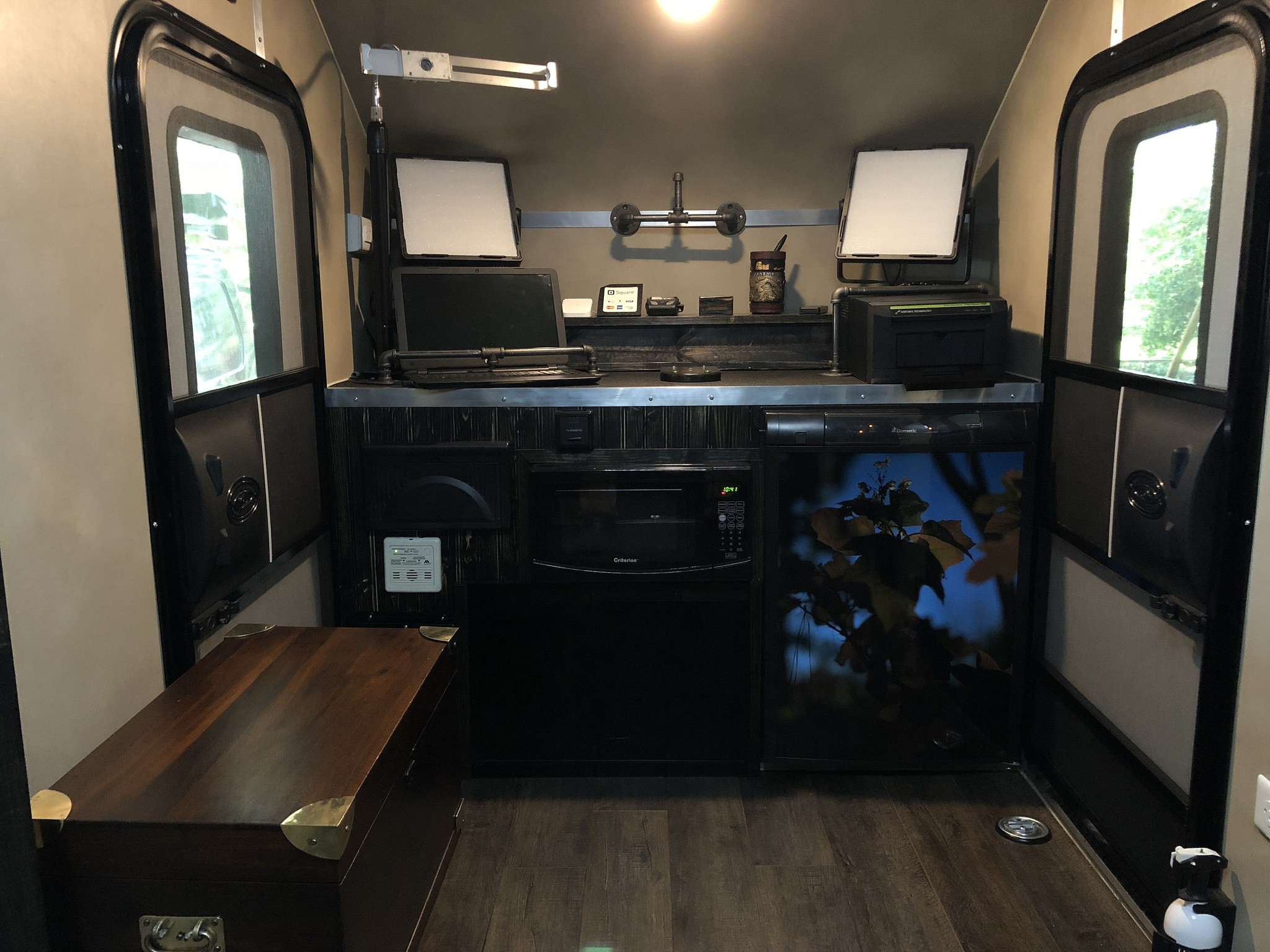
[185, 243, 257, 393]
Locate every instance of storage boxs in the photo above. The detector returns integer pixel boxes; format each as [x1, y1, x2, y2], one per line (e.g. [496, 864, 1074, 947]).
[31, 625, 467, 952]
